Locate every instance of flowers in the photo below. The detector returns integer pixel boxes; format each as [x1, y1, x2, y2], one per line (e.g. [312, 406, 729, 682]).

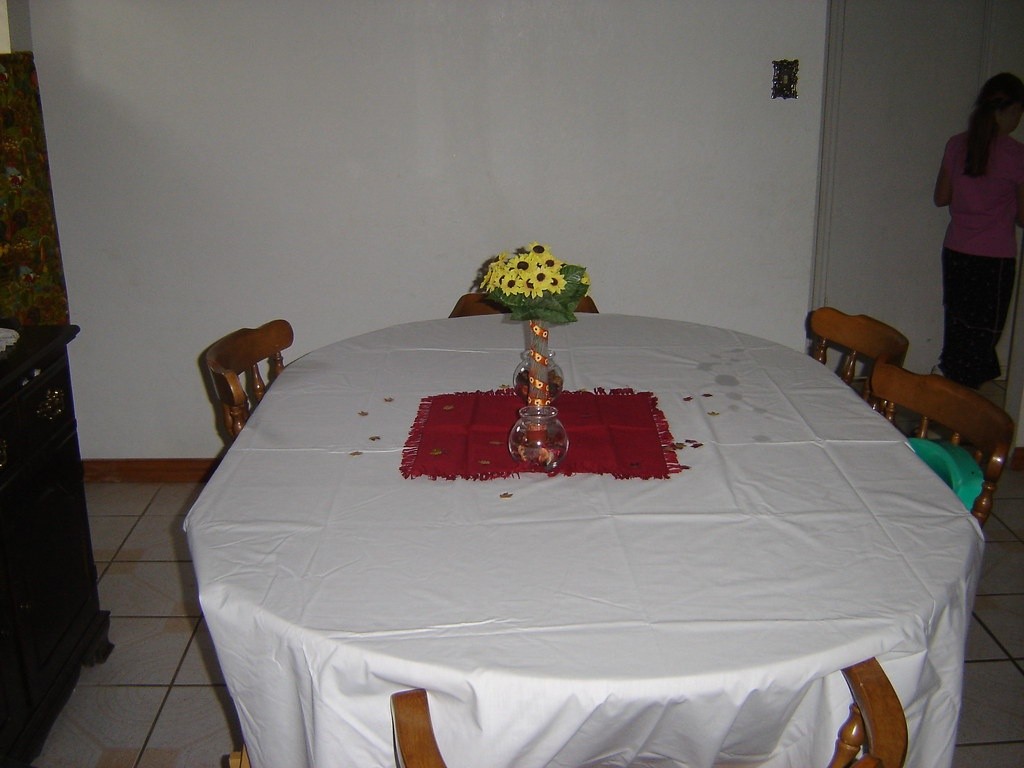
[473, 242, 591, 409]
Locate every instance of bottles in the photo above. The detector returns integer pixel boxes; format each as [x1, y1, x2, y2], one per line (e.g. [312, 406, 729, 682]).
[508, 406, 568, 470]
[513, 349, 564, 403]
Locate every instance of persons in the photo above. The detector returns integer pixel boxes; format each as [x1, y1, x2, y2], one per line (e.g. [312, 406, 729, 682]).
[930, 73, 1024, 391]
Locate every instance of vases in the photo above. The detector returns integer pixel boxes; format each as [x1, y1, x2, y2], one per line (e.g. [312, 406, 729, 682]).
[512, 349, 564, 406]
[508, 407, 568, 469]
[527, 320, 549, 450]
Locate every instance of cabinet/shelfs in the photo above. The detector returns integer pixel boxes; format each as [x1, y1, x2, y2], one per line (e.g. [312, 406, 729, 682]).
[0, 314, 115, 768]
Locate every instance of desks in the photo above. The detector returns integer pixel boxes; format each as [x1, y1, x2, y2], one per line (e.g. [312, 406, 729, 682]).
[181, 314, 988, 768]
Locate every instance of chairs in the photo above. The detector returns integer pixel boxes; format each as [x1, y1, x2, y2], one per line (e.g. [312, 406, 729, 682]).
[391, 657, 909, 768]
[199, 319, 295, 449]
[810, 308, 909, 415]
[869, 362, 1013, 528]
[448, 293, 599, 318]
